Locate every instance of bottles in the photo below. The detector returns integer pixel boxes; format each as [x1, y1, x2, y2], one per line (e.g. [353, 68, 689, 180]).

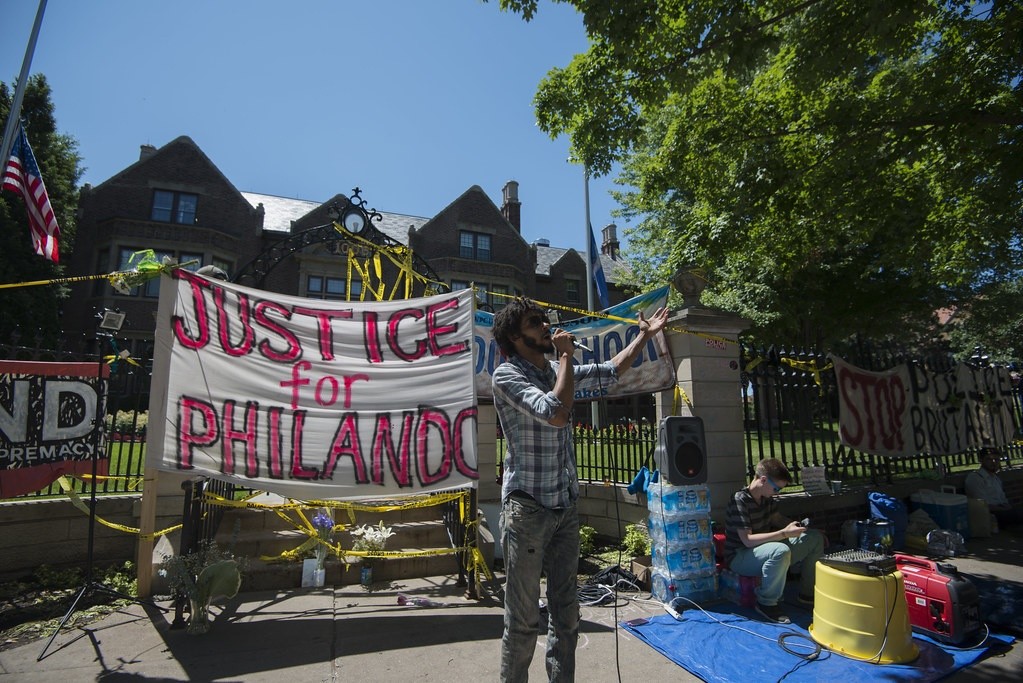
[647, 481, 762, 606]
[991, 514, 998, 533]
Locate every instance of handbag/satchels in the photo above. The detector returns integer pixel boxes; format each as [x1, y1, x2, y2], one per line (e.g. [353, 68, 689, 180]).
[906, 509, 939, 551]
[868, 491, 908, 549]
[850, 507, 895, 554]
[927, 527, 967, 557]
[967, 497, 990, 538]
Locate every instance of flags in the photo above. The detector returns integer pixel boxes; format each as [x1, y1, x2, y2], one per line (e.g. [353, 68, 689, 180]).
[590, 228, 609, 308]
[2, 125, 61, 263]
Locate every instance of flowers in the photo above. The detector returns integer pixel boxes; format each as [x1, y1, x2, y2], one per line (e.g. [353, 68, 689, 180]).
[157, 537, 251, 627]
[312, 513, 337, 560]
[349, 520, 397, 566]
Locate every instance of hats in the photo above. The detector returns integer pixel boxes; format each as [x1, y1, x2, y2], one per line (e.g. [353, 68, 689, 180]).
[979, 447, 1000, 456]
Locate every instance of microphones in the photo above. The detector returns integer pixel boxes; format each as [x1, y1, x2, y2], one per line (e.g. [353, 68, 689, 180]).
[549, 328, 593, 353]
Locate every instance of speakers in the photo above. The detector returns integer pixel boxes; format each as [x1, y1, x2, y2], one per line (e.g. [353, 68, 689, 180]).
[654, 416, 708, 487]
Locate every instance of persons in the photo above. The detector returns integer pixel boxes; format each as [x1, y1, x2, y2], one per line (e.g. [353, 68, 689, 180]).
[724, 459, 824, 623]
[964, 449, 1023, 531]
[492, 297, 669, 683]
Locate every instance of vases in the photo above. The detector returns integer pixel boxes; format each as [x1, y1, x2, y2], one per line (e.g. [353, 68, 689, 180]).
[323, 555, 340, 587]
[186, 597, 212, 636]
[361, 560, 374, 586]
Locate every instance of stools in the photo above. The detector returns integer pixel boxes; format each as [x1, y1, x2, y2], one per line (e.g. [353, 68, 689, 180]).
[719, 572, 760, 608]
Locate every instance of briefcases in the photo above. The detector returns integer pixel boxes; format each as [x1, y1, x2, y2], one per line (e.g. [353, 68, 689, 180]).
[910, 484, 967, 541]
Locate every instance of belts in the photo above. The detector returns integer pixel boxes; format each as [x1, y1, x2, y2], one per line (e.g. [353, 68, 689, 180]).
[509, 488, 572, 500]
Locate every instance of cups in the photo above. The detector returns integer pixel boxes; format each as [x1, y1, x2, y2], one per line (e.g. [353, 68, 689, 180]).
[831, 480, 843, 495]
[361, 566, 372, 584]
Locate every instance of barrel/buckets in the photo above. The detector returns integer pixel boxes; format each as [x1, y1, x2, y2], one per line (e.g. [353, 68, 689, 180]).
[808, 561, 921, 665]
[968, 498, 991, 538]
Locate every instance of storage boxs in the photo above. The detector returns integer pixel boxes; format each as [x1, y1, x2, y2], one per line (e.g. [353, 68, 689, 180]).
[629, 555, 653, 584]
[909, 489, 970, 544]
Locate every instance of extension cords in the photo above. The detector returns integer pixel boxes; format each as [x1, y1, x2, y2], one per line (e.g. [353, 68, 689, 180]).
[664, 603, 684, 621]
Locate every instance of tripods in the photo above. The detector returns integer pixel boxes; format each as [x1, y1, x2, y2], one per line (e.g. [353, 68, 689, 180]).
[36, 346, 169, 661]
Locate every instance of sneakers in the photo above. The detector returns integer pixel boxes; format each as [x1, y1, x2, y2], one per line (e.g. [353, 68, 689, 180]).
[797, 592, 814, 604]
[754, 600, 790, 623]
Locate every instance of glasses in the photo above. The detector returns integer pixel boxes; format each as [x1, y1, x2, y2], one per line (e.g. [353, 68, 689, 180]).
[768, 479, 781, 494]
[517, 315, 550, 327]
